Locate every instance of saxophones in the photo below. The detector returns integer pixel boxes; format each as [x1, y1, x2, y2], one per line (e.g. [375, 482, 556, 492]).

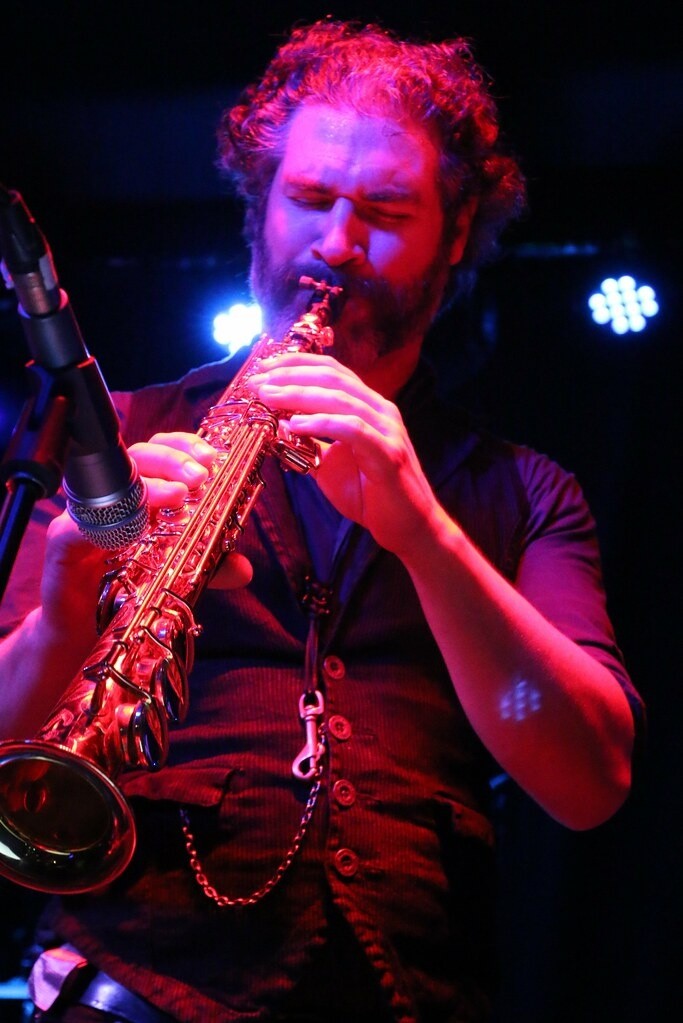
[0, 275, 345, 897]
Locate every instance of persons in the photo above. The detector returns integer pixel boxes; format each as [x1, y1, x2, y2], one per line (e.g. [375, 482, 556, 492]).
[0, 17, 649, 1023]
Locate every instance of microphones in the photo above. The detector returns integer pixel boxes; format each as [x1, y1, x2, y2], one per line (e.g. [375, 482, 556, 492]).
[0, 189, 146, 549]
